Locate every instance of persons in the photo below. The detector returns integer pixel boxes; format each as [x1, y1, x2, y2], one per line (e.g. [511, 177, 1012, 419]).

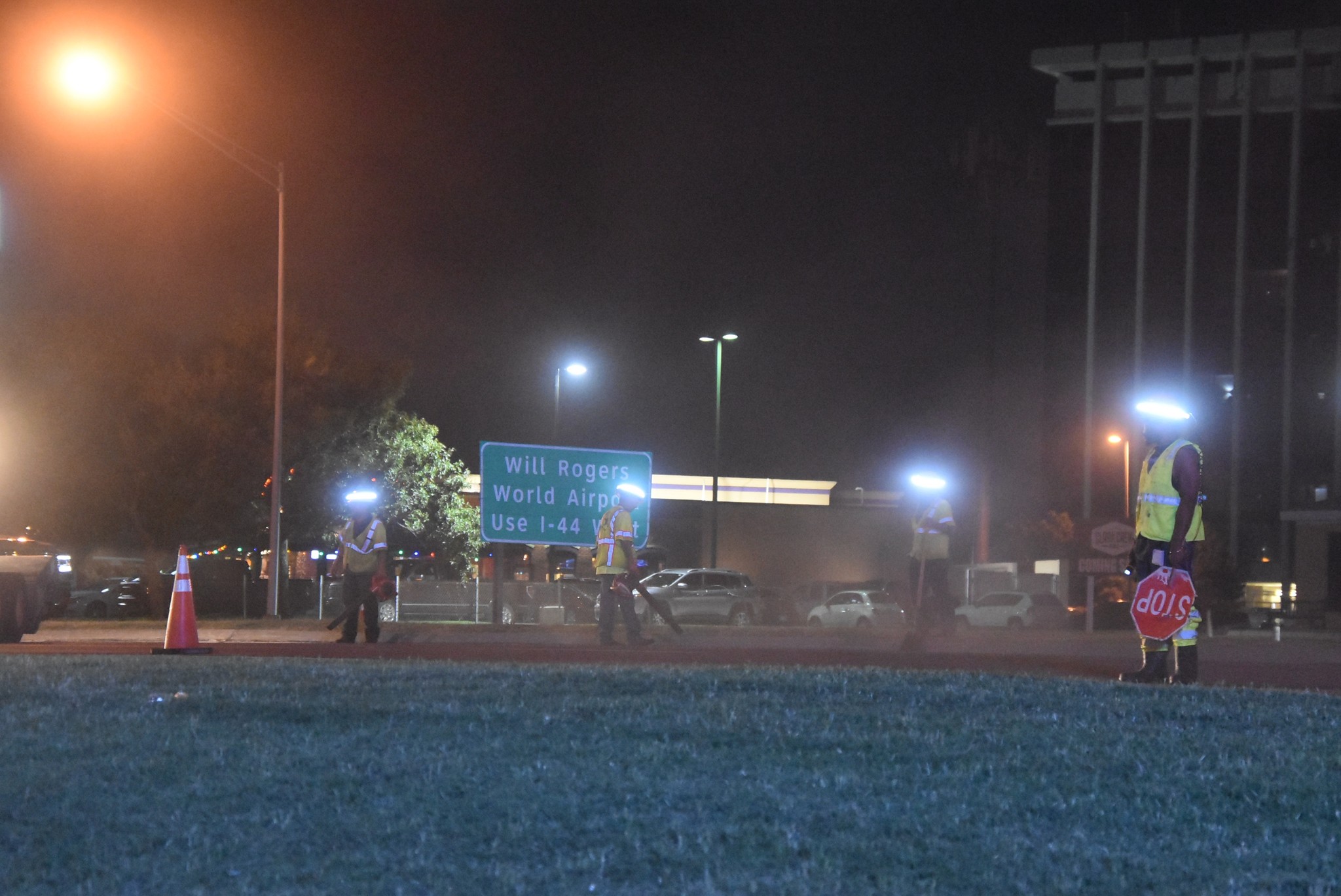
[330, 500, 388, 644]
[595, 492, 655, 648]
[1123, 414, 1206, 682]
[904, 473, 957, 640]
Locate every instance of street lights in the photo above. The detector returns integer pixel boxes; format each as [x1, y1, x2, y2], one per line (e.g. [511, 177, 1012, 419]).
[56, 42, 287, 624]
[1107, 431, 1131, 521]
[691, 329, 742, 571]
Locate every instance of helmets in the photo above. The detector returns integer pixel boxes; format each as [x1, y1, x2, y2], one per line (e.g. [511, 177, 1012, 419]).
[610, 573, 633, 598]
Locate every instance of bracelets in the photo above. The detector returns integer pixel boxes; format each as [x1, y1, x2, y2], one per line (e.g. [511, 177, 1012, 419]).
[1169, 547, 1183, 554]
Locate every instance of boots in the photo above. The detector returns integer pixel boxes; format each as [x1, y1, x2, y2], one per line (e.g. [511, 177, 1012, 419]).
[1173, 646, 1197, 686]
[1121, 650, 1170, 684]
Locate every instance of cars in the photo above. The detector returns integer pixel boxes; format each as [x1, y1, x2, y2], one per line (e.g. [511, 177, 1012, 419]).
[1, 536, 627, 644]
[953, 590, 1070, 631]
[593, 568, 762, 625]
[806, 588, 907, 629]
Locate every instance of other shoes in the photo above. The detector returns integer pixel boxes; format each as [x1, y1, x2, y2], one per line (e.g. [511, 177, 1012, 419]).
[364, 628, 380, 643]
[626, 631, 654, 647]
[336, 635, 356, 643]
[599, 634, 617, 647]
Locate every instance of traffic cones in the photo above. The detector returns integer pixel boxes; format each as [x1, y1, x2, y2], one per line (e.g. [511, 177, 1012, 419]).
[551, 360, 588, 447]
[151, 542, 214, 658]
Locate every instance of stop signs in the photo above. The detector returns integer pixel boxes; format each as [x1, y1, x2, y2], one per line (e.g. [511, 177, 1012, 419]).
[1128, 566, 1199, 641]
